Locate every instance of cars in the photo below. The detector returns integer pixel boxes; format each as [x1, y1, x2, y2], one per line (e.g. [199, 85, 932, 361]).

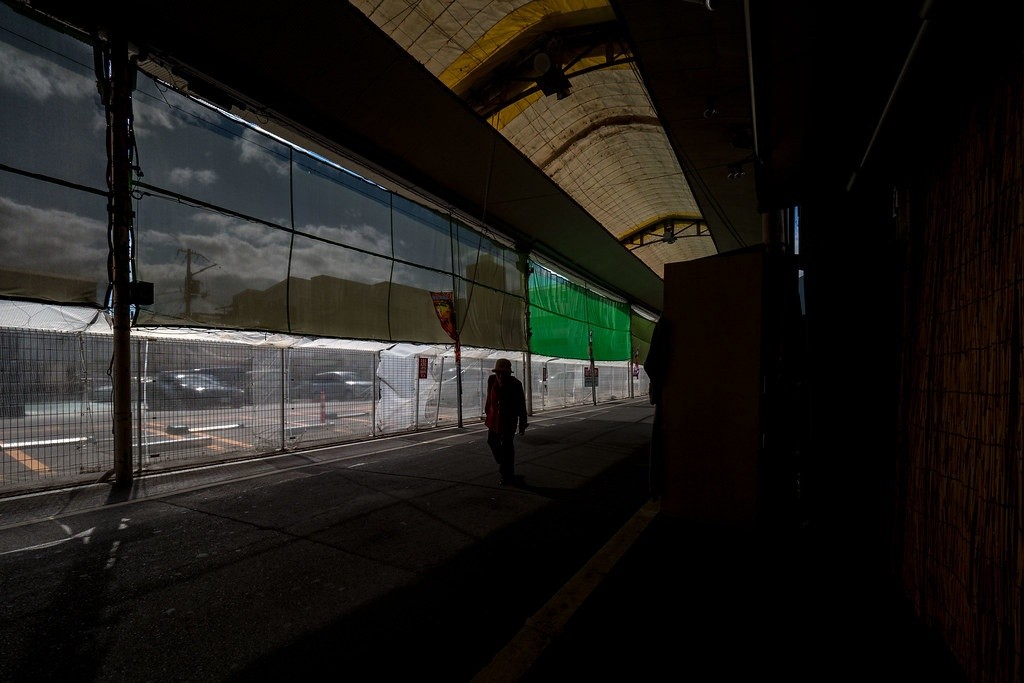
[143, 374, 245, 411]
[430, 367, 494, 406]
[92, 377, 149, 403]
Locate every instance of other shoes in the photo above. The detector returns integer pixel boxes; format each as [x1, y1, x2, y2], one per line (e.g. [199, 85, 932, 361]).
[498, 477, 511, 485]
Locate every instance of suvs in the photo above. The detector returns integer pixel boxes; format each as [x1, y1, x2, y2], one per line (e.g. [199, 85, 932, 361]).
[538, 370, 584, 395]
[189, 367, 379, 401]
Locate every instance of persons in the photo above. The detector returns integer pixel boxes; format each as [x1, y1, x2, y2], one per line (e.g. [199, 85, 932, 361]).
[484, 359, 530, 485]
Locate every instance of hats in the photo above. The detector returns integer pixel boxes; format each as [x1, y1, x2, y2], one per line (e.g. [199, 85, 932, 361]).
[492, 359, 514, 373]
